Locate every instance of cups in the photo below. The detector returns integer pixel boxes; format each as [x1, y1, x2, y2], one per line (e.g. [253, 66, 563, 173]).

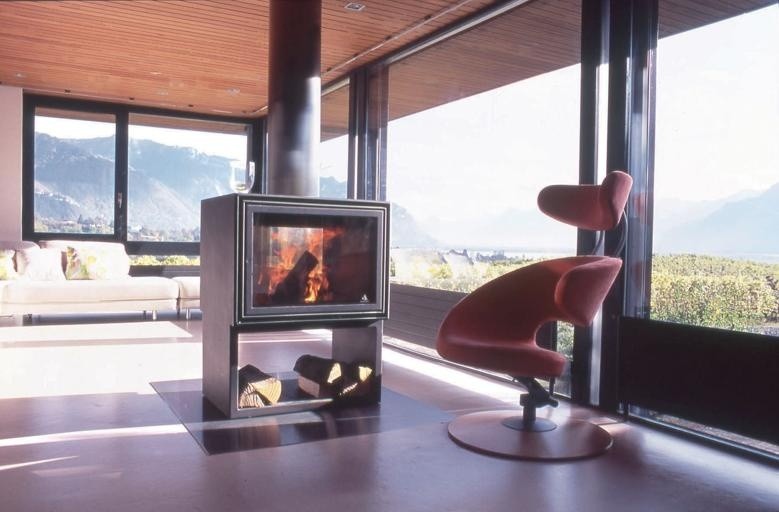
[230, 160, 256, 192]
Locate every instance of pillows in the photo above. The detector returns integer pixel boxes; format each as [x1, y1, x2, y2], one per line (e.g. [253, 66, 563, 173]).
[0, 245, 104, 280]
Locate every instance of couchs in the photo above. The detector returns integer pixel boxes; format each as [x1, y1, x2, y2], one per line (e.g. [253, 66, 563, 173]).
[0, 240, 179, 322]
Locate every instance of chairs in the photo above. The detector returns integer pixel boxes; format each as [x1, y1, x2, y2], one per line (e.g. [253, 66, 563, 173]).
[436, 170, 634, 461]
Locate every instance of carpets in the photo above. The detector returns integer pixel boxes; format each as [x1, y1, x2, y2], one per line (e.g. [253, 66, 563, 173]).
[149, 371, 453, 456]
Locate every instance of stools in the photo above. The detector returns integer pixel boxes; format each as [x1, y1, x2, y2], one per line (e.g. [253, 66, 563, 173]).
[172, 275, 200, 319]
